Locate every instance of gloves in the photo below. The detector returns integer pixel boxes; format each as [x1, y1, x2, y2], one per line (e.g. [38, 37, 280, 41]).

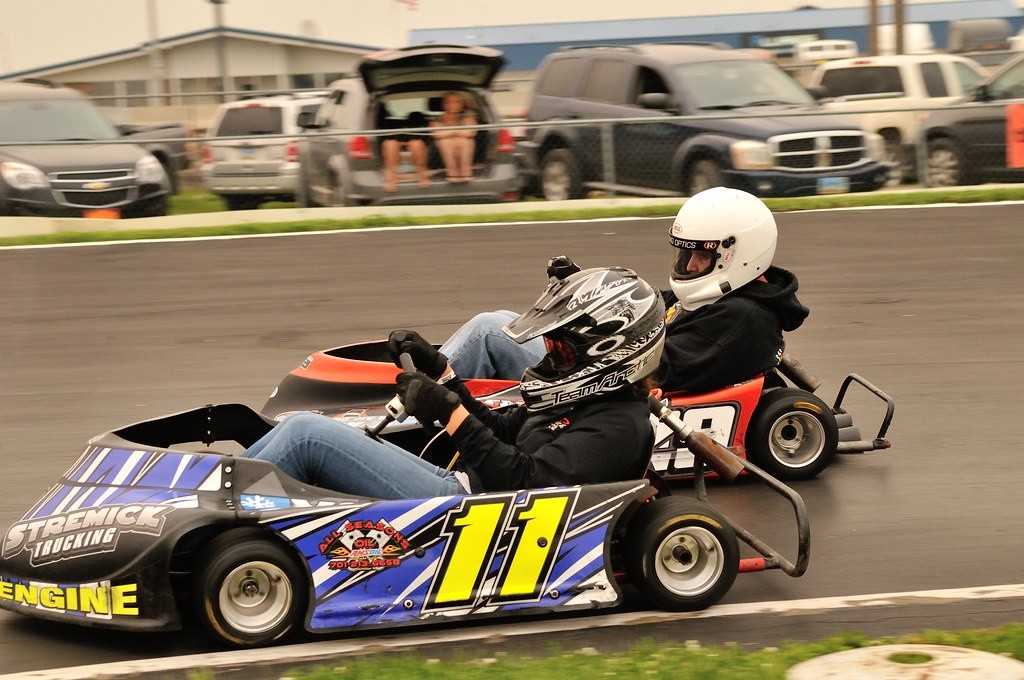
[546, 255, 582, 281]
[387, 330, 448, 383]
[395, 369, 461, 426]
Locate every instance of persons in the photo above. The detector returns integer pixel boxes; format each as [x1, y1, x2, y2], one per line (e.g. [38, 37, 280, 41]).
[379, 111, 433, 193]
[429, 91, 479, 183]
[232, 265, 666, 507]
[436, 186, 811, 391]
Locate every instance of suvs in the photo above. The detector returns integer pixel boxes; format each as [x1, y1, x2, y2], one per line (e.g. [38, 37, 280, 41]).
[295, 39, 529, 208]
[199, 87, 329, 210]
[0, 77, 176, 219]
[522, 40, 893, 200]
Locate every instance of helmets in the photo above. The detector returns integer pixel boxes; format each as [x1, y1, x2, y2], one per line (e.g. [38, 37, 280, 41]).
[669, 187, 778, 311]
[502, 266, 666, 417]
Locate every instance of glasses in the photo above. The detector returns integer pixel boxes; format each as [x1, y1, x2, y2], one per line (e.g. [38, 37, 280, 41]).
[543, 336, 579, 371]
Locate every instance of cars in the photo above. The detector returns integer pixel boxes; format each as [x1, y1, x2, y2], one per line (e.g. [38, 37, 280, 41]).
[804, 54, 994, 178]
[893, 51, 1023, 187]
